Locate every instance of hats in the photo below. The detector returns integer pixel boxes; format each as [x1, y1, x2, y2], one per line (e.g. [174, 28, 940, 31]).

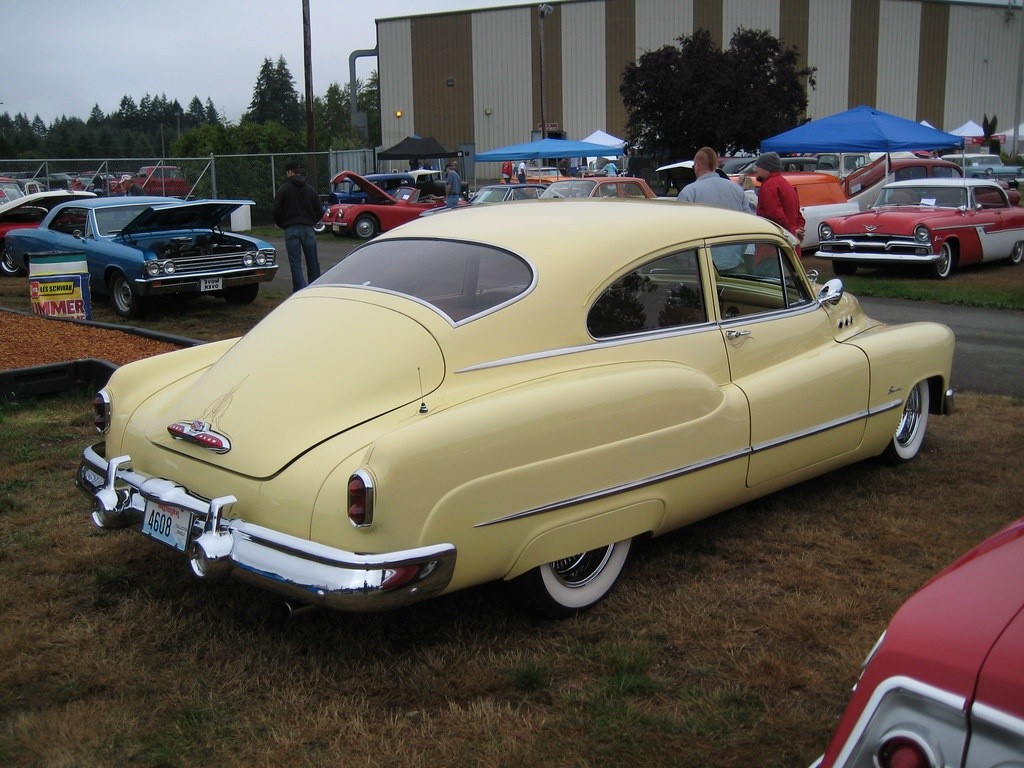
[755, 152, 783, 172]
[285, 161, 301, 174]
[119, 175, 132, 184]
[444, 164, 452, 171]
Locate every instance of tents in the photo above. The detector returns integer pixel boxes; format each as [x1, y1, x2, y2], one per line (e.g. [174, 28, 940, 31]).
[580, 130, 629, 147]
[377, 135, 464, 168]
[474, 138, 623, 181]
[759, 104, 964, 204]
[918, 119, 1024, 154]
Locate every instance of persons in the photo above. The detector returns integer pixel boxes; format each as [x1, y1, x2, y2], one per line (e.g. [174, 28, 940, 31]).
[121, 175, 146, 196]
[755, 152, 805, 262]
[931, 150, 942, 160]
[273, 161, 323, 292]
[599, 160, 619, 177]
[445, 160, 462, 207]
[676, 147, 751, 275]
[517, 160, 528, 184]
[502, 161, 513, 184]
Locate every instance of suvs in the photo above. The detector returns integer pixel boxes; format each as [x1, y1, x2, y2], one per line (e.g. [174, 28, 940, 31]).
[138, 166, 189, 197]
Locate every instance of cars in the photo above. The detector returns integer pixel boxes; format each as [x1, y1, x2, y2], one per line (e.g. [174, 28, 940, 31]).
[312, 172, 416, 236]
[652, 150, 1024, 253]
[0, 170, 137, 278]
[418, 183, 565, 218]
[807, 516, 1024, 768]
[814, 178, 1023, 281]
[0, 197, 279, 321]
[539, 176, 679, 202]
[73, 198, 957, 622]
[501, 164, 577, 186]
[321, 169, 467, 240]
